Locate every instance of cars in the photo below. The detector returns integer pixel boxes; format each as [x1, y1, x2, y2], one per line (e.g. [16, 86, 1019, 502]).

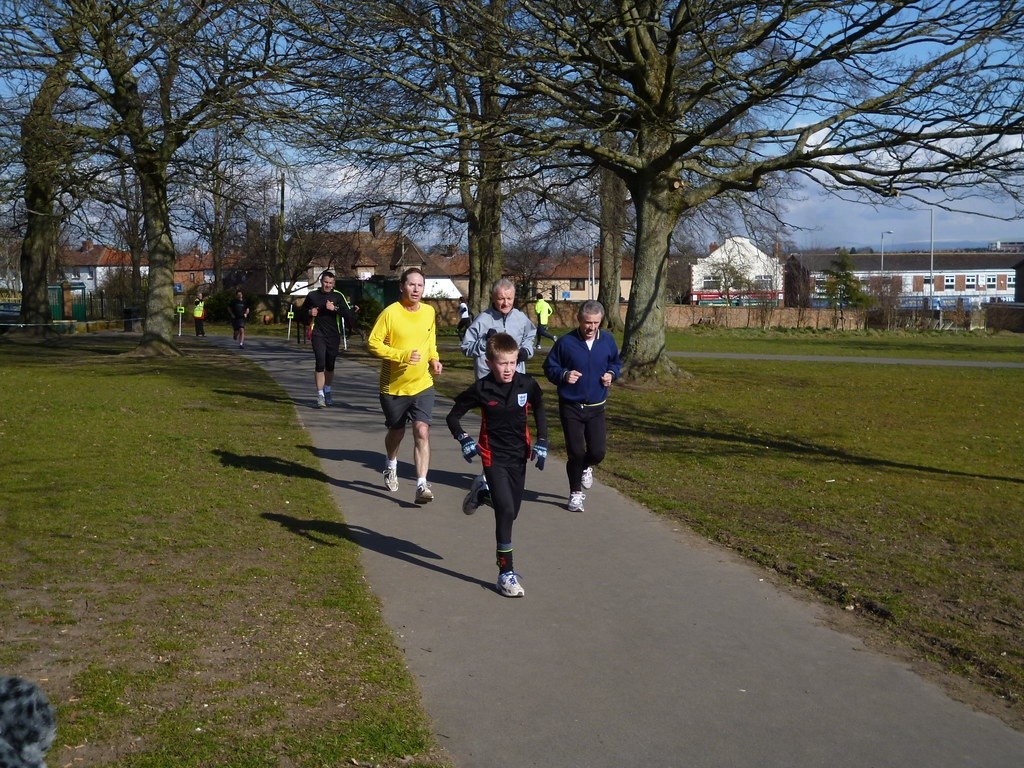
[0, 302, 22, 334]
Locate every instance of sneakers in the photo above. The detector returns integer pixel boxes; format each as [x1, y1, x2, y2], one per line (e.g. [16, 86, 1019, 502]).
[462, 476, 490, 515]
[496, 571, 525, 597]
[582, 467, 593, 488]
[384, 466, 399, 491]
[325, 392, 333, 406]
[414, 484, 433, 504]
[568, 492, 585, 513]
[317, 394, 326, 407]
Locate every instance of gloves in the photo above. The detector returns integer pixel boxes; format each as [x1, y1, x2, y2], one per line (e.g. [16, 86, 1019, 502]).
[458, 434, 481, 464]
[531, 440, 548, 470]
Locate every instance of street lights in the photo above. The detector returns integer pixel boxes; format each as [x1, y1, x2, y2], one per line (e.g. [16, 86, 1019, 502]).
[880, 230, 894, 277]
[907, 207, 933, 310]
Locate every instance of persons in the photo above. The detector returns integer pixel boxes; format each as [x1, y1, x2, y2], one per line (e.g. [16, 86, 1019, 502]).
[193, 292, 206, 338]
[542, 299, 619, 512]
[298, 271, 353, 408]
[447, 334, 547, 599]
[458, 297, 472, 340]
[533, 292, 557, 350]
[367, 268, 442, 503]
[464, 278, 538, 376]
[228, 290, 250, 350]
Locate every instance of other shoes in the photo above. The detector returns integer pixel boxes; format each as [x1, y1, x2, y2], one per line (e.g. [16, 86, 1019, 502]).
[552, 335, 557, 342]
[535, 344, 542, 349]
